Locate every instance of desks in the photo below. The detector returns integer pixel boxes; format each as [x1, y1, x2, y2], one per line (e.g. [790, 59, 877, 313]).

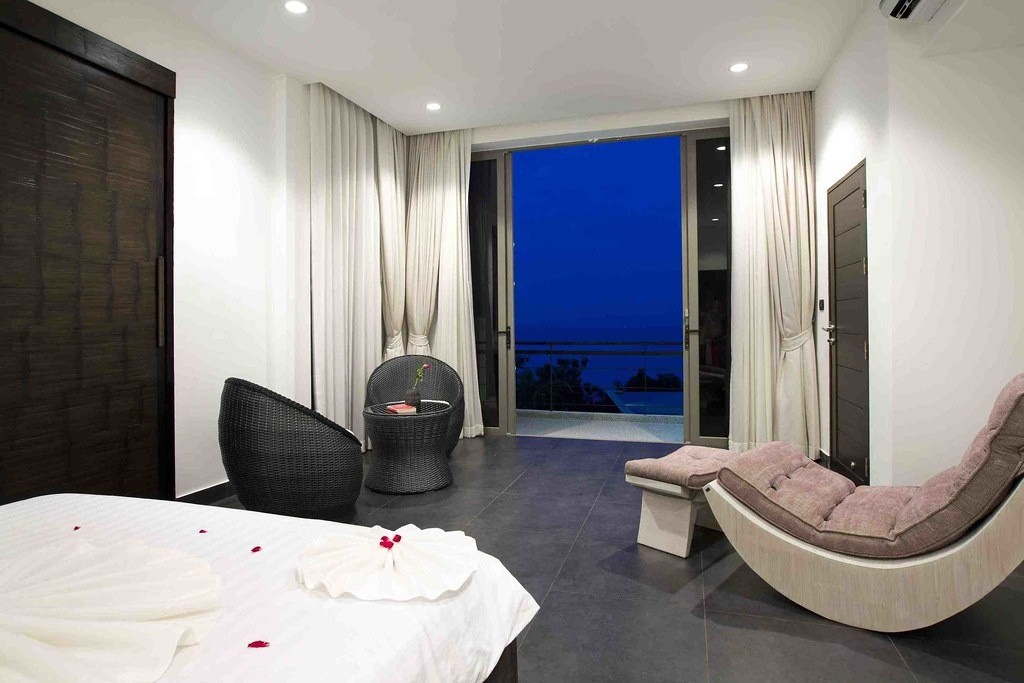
[363, 400, 454, 494]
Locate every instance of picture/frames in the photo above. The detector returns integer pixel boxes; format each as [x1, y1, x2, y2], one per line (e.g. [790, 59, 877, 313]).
[405, 389, 421, 406]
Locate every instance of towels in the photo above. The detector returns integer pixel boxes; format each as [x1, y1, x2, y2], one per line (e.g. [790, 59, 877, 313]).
[0, 538, 228, 683]
[292, 522, 480, 603]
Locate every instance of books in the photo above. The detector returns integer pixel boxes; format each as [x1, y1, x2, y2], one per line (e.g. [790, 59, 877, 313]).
[387, 404, 417, 413]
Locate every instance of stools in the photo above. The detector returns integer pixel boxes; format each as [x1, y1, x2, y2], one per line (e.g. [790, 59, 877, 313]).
[624, 444, 739, 558]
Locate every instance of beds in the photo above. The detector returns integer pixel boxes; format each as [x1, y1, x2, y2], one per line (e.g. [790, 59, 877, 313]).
[0, 493, 541, 683]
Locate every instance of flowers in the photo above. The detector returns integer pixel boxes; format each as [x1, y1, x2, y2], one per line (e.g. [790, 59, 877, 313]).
[413, 363, 430, 387]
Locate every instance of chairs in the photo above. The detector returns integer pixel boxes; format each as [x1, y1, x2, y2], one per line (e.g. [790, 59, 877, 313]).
[703, 373, 1024, 633]
[365, 355, 465, 455]
[218, 378, 363, 522]
